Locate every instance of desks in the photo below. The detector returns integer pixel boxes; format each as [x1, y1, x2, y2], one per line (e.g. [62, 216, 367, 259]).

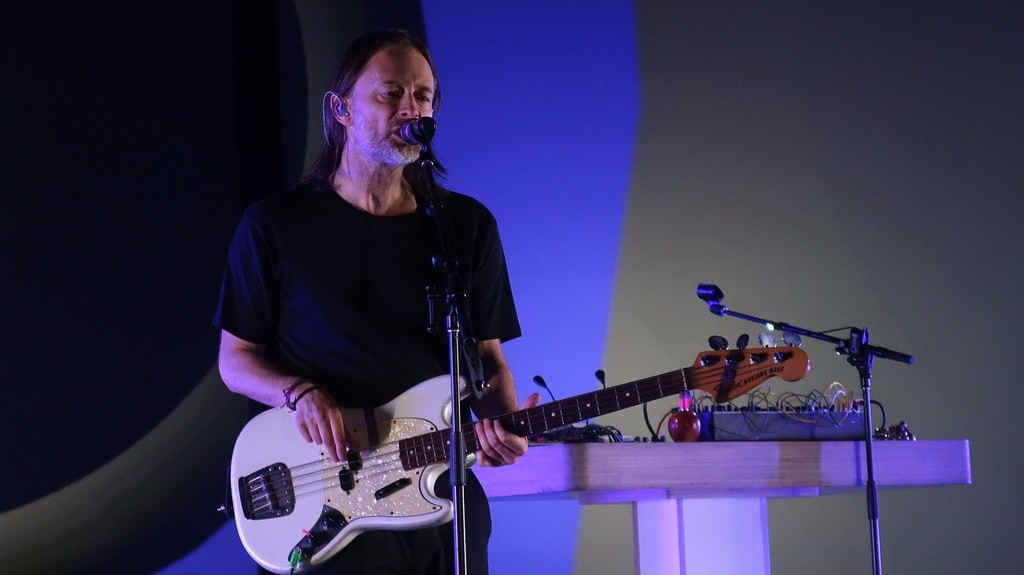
[472, 440, 973, 575]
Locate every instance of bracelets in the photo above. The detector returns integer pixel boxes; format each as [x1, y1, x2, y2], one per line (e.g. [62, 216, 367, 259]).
[281, 377, 309, 409]
[293, 385, 319, 411]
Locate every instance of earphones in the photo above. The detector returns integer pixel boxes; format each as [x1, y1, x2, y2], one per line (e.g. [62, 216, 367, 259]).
[337, 103, 350, 116]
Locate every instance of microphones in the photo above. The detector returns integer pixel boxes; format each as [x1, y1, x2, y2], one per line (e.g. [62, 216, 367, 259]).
[401, 116, 437, 146]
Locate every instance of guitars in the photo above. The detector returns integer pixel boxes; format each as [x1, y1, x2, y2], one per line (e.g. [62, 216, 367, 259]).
[230, 325, 810, 575]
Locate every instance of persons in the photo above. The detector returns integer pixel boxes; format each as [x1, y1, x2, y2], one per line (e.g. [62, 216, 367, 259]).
[213, 27, 540, 575]
